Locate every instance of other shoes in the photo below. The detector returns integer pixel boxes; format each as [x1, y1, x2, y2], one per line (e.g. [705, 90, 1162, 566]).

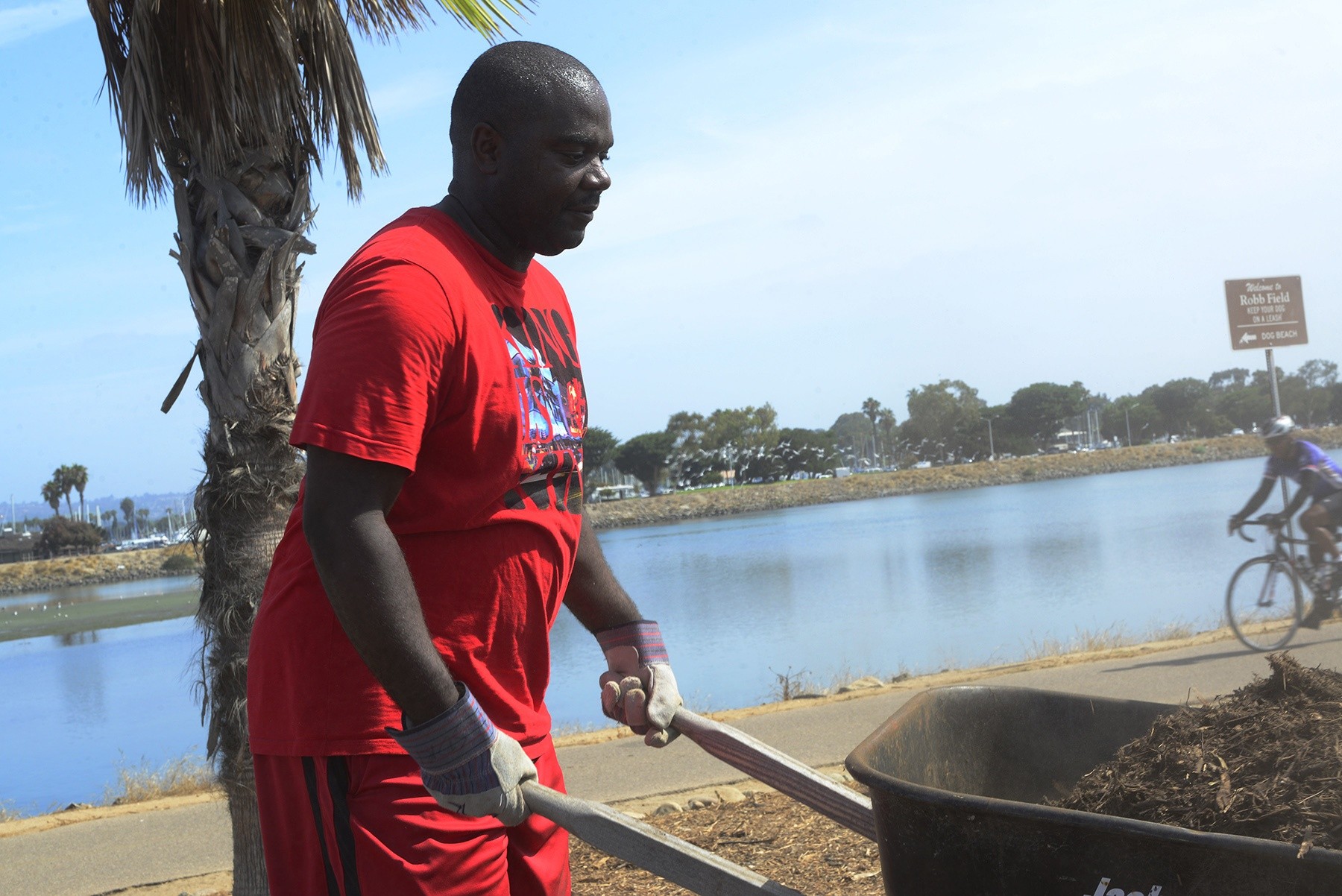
[1300, 604, 1332, 629]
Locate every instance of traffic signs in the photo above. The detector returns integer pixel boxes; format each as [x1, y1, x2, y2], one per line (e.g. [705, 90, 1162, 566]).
[1224, 271, 1310, 351]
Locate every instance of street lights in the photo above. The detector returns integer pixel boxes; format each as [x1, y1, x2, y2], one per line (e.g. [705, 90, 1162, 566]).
[1123, 401, 1142, 448]
[978, 414, 1001, 459]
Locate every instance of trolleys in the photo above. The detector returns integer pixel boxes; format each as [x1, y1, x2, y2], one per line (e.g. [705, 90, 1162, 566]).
[519, 664, 1342, 896]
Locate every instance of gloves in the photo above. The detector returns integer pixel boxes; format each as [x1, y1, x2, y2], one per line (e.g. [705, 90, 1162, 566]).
[384, 680, 538, 827]
[600, 620, 684, 749]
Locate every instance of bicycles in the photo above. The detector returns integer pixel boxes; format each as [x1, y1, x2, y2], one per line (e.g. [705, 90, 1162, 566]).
[1226, 517, 1342, 652]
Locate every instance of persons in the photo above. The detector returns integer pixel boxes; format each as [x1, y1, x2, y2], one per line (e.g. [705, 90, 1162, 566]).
[247, 42, 682, 896]
[1227, 414, 1342, 629]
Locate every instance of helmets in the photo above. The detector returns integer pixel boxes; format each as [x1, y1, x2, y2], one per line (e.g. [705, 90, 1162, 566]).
[1259, 415, 1299, 442]
